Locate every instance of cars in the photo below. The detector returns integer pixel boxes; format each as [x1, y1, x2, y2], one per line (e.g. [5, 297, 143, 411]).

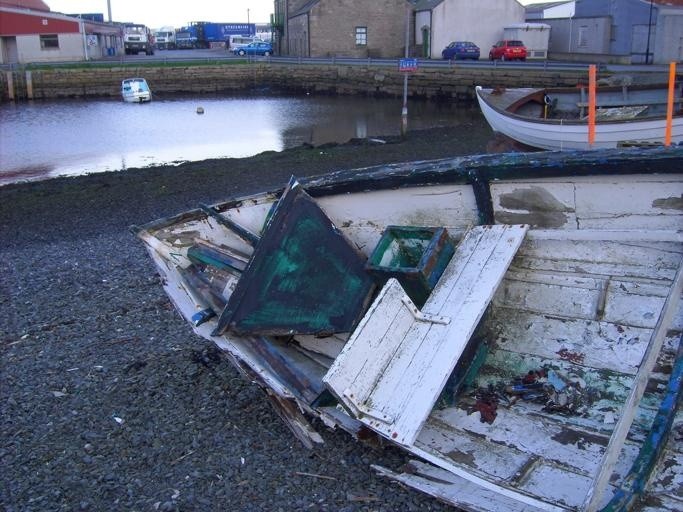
[489, 40, 527, 62]
[225, 35, 273, 56]
[442, 41, 480, 61]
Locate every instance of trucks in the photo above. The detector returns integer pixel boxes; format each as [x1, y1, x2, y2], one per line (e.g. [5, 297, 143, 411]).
[123, 25, 154, 55]
[156, 22, 255, 50]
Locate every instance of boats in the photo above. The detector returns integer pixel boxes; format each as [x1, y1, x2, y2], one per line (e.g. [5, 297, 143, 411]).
[121, 78, 152, 103]
